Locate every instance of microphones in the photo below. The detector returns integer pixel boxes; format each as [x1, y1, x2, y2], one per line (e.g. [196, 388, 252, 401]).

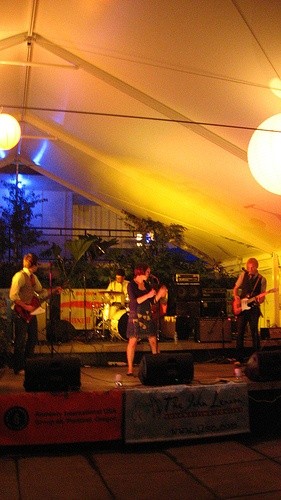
[242, 267, 249, 274]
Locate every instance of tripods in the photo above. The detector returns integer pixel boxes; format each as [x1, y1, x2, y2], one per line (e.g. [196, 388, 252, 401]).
[89, 291, 121, 341]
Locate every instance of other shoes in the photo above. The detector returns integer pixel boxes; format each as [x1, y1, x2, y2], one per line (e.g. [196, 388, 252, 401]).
[18, 370, 25, 376]
[127, 372, 133, 376]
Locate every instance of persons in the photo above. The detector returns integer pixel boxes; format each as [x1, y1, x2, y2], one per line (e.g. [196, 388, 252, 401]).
[126, 263, 165, 378]
[8, 252, 62, 374]
[105, 269, 130, 311]
[232, 258, 267, 366]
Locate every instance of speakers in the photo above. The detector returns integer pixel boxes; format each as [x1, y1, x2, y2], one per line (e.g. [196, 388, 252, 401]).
[137, 353, 193, 386]
[23, 355, 81, 392]
[159, 282, 233, 343]
[245, 350, 281, 382]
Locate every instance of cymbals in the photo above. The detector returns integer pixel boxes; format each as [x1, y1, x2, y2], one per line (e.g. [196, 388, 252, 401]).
[99, 290, 124, 294]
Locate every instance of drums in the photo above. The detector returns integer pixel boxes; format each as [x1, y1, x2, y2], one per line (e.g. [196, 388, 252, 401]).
[110, 310, 141, 343]
[103, 302, 122, 321]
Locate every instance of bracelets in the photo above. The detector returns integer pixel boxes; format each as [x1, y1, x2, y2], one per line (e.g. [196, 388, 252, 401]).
[155, 297, 158, 303]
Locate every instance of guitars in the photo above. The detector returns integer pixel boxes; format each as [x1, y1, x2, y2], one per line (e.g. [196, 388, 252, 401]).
[14, 286, 63, 322]
[232, 288, 279, 315]
